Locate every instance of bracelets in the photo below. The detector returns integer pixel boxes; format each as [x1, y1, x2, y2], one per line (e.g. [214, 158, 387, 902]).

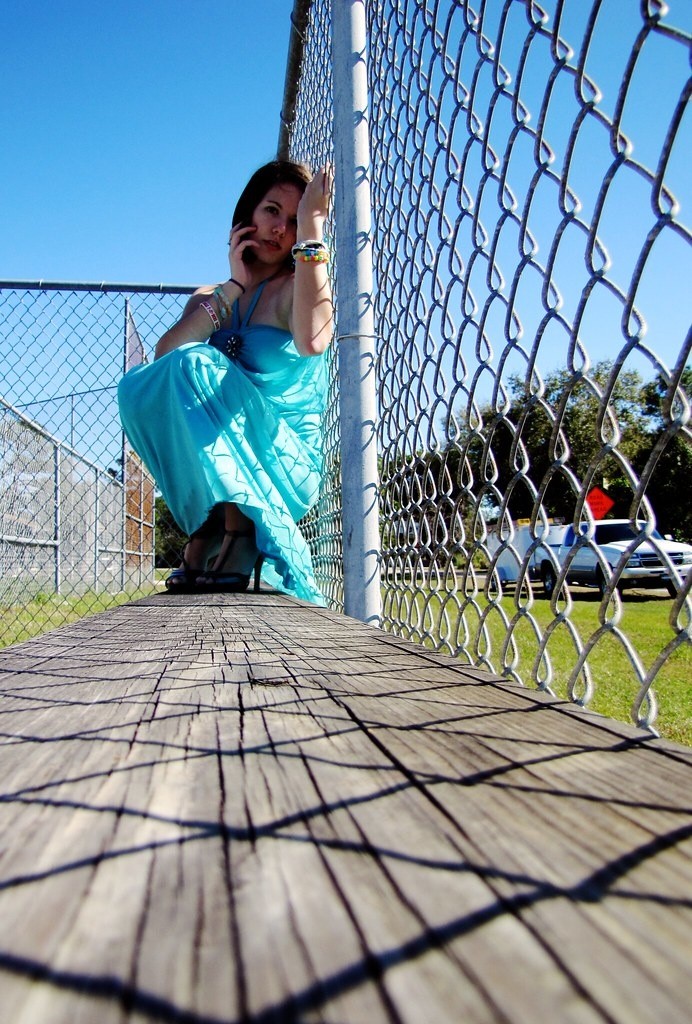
[290, 240, 328, 264]
[201, 285, 231, 330]
[229, 279, 248, 294]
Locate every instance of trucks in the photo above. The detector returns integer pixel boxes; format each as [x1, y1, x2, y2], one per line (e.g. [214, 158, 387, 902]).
[486, 519, 692, 599]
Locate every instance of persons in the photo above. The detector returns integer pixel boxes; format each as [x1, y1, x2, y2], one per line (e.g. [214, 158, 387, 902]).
[118, 159, 334, 594]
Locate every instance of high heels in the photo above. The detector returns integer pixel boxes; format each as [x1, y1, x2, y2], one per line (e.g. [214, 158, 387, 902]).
[164, 519, 225, 593]
[192, 526, 264, 594]
